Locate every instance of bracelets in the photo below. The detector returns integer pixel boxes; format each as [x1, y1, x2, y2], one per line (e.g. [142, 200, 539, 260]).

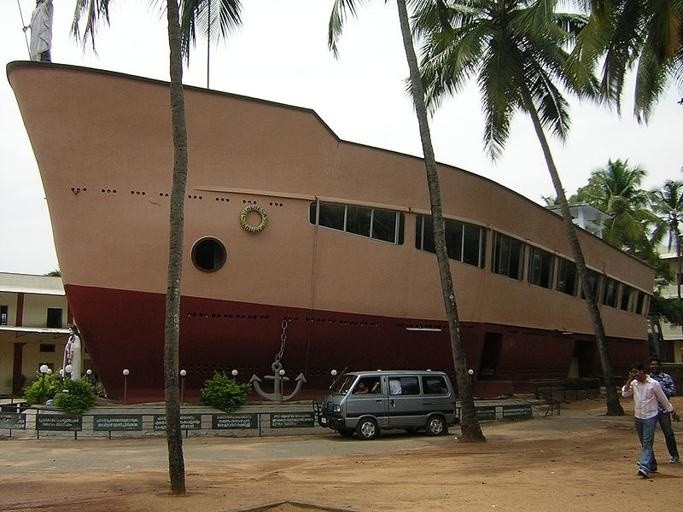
[671, 411, 675, 415]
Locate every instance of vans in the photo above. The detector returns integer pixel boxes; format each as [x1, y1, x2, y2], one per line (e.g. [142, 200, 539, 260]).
[317, 370, 457, 440]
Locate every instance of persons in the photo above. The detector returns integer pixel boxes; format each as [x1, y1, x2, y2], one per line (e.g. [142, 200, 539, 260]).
[634, 355, 680, 467]
[619, 361, 680, 478]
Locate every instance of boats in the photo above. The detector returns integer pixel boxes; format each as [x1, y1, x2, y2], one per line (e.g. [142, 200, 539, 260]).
[6, 59, 658, 406]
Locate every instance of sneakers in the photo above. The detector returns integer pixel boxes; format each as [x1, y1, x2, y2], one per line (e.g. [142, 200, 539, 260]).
[635, 455, 680, 478]
